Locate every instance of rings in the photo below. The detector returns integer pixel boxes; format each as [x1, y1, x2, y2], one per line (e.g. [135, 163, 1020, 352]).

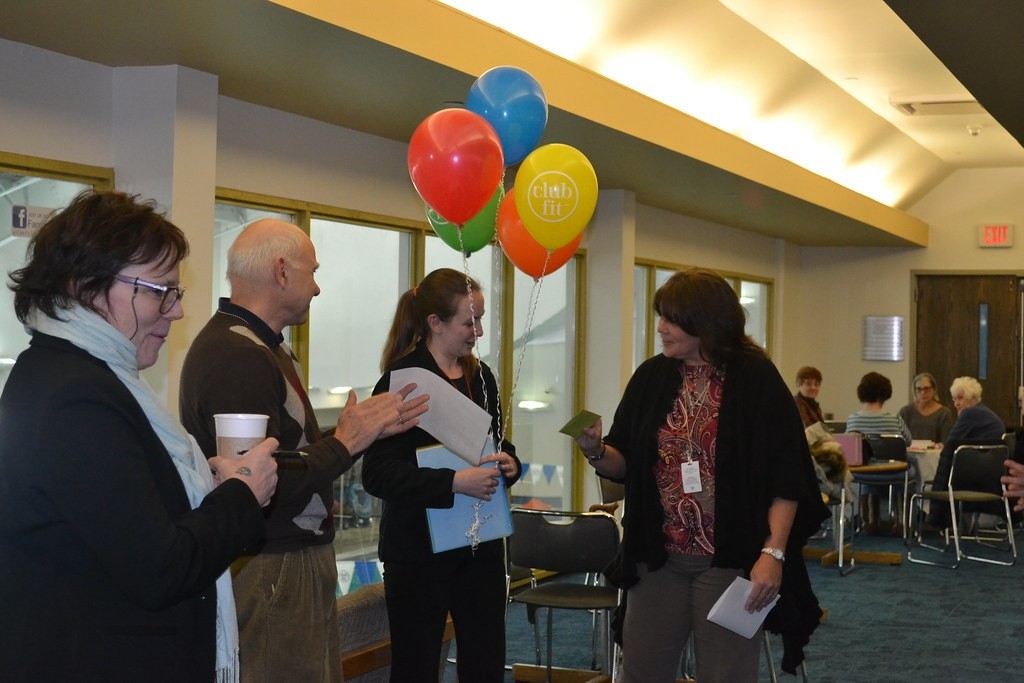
[768, 593, 772, 598]
[398, 415, 403, 424]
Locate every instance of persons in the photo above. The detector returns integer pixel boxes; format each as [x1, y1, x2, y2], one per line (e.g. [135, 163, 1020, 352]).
[576, 268, 834, 683]
[846, 371, 916, 534]
[0, 191, 280, 683]
[180, 218, 430, 683]
[362, 269, 521, 683]
[896, 373, 953, 450]
[1000, 460, 1024, 511]
[792, 366, 854, 502]
[923, 377, 1006, 535]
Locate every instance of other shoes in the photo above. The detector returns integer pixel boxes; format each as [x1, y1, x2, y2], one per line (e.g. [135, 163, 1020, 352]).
[995, 522, 1020, 533]
[923, 522, 939, 531]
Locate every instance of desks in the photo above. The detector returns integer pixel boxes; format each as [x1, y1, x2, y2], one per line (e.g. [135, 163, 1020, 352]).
[862, 447, 944, 531]
[804, 459, 910, 566]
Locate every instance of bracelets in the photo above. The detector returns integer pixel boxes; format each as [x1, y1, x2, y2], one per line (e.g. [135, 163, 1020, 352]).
[582, 441, 606, 460]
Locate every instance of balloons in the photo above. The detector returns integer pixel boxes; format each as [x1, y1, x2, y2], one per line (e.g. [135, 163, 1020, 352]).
[406, 66, 598, 282]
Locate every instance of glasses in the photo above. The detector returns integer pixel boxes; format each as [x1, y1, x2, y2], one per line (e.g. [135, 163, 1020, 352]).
[113, 274, 186, 314]
[915, 386, 932, 393]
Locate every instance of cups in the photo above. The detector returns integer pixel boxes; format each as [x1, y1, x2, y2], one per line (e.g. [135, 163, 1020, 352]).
[213, 413, 270, 462]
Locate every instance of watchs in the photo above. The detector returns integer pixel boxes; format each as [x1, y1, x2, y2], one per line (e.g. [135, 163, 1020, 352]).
[762, 547, 785, 562]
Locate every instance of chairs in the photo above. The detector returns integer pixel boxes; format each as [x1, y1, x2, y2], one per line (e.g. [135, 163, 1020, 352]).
[816, 478, 855, 575]
[851, 430, 1019, 568]
[504, 473, 809, 683]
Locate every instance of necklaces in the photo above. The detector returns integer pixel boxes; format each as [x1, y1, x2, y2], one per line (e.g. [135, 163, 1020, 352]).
[684, 377, 709, 416]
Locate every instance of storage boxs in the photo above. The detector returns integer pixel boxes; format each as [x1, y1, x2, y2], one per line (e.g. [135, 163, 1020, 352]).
[830, 432, 861, 467]
